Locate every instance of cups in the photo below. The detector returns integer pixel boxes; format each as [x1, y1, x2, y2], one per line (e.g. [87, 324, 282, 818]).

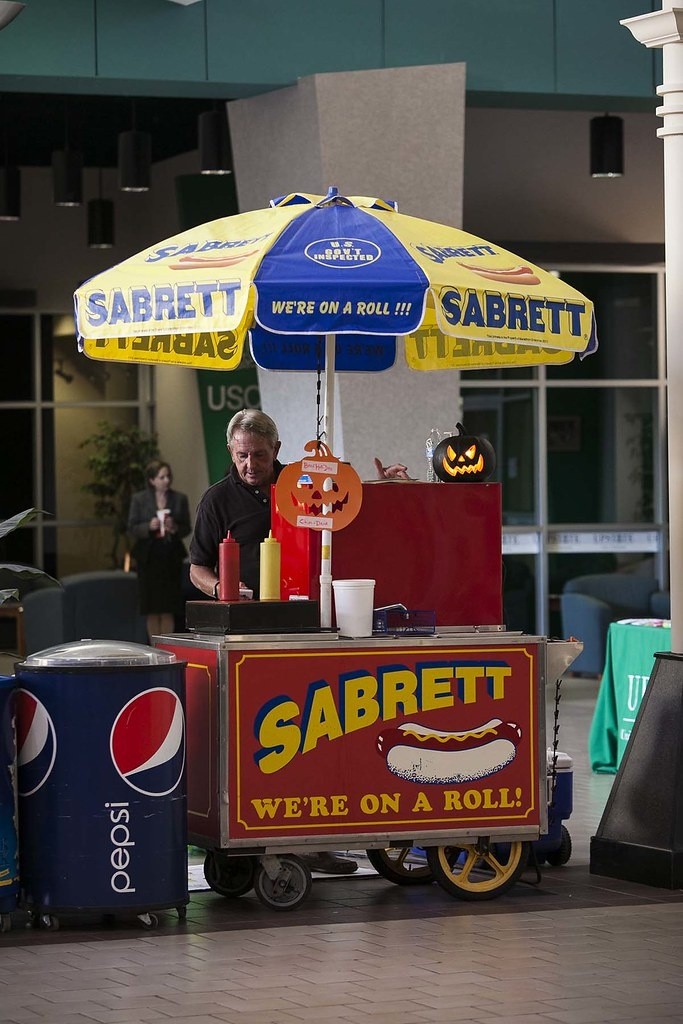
[157, 508, 172, 536]
[332, 579, 376, 637]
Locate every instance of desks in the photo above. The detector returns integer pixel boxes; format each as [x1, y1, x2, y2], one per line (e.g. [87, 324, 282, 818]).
[589, 618, 671, 774]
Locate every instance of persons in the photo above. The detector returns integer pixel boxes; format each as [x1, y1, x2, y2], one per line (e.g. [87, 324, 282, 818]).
[190, 409, 410, 599]
[126, 461, 193, 644]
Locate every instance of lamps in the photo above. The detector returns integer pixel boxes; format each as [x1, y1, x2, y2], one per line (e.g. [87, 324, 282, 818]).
[199, 98, 232, 175]
[118, 98, 152, 192]
[86, 168, 116, 248]
[0, 164, 21, 223]
[51, 94, 83, 207]
[54, 358, 75, 383]
[589, 111, 625, 179]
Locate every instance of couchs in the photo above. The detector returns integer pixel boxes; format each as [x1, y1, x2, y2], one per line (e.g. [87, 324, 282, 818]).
[560, 575, 669, 679]
[14, 570, 138, 654]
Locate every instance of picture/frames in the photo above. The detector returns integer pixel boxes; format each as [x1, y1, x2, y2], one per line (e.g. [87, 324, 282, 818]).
[547, 413, 584, 454]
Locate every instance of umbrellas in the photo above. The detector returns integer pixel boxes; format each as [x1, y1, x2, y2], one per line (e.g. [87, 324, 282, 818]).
[73, 186, 598, 625]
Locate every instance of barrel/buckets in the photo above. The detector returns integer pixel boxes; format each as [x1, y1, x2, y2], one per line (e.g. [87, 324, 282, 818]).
[0, 675, 21, 913]
[14, 661, 190, 914]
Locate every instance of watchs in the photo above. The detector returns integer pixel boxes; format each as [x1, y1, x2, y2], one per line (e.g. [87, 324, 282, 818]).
[213, 581, 220, 599]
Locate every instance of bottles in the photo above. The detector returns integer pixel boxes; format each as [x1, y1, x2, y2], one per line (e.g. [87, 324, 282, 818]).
[259, 529, 282, 600]
[426, 429, 442, 482]
[219, 530, 240, 601]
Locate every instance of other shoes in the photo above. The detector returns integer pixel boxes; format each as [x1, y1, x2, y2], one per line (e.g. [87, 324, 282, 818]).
[307, 854, 359, 874]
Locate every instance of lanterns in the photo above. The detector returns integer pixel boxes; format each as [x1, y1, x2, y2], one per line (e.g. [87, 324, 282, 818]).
[433, 435, 496, 482]
